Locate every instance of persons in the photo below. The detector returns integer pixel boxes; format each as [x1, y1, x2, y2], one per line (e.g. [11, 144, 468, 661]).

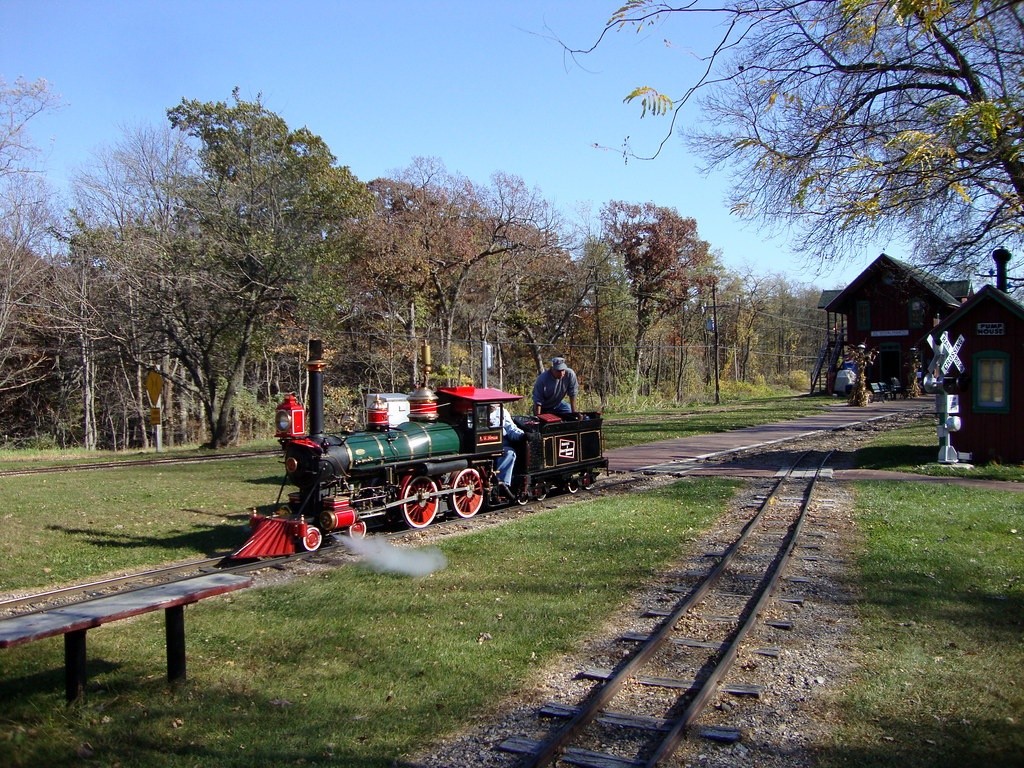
[490, 407, 536, 501]
[533, 357, 584, 422]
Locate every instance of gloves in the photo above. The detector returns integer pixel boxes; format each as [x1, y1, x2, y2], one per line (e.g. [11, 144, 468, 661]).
[523, 432, 534, 442]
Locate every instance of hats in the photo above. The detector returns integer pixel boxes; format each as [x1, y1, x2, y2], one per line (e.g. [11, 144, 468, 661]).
[552, 358, 566, 370]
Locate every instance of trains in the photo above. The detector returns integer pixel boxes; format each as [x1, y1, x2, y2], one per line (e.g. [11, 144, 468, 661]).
[228, 336, 611, 566]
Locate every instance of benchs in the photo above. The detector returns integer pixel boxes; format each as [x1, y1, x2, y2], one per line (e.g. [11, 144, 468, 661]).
[870, 382, 895, 403]
[0, 571, 253, 708]
